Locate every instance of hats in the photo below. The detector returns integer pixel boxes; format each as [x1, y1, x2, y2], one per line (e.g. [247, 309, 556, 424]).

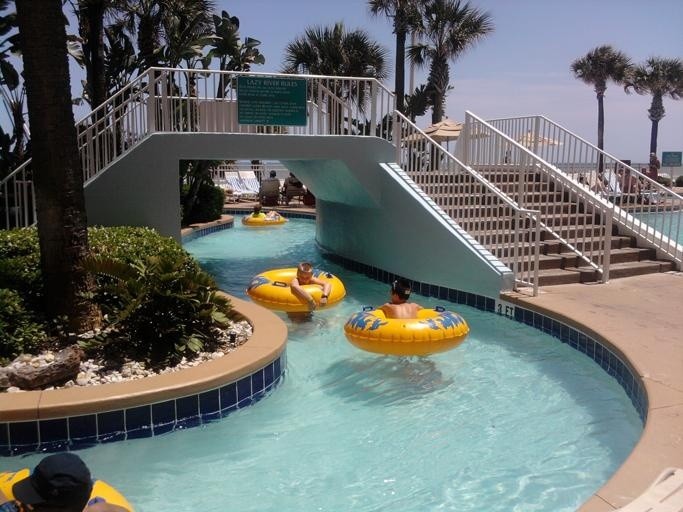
[12, 452, 92, 504]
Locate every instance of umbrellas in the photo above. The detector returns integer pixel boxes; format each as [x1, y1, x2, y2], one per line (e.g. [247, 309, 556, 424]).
[401, 115, 491, 169]
[511, 129, 562, 147]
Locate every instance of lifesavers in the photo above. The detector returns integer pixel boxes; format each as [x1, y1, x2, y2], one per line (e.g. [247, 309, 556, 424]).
[241, 214, 285, 227]
[246, 267, 347, 313]
[344, 306, 470, 357]
[0, 470, 135, 511]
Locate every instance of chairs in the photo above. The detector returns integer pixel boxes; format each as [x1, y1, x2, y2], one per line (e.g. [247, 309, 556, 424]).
[570, 168, 665, 205]
[224, 170, 306, 206]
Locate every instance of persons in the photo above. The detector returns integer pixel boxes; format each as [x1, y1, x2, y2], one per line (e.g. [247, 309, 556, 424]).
[285, 262, 332, 322]
[280, 172, 299, 205]
[618, 152, 663, 204]
[259, 170, 280, 190]
[577, 172, 610, 194]
[377, 278, 423, 320]
[0, 452, 128, 512]
[250, 203, 289, 221]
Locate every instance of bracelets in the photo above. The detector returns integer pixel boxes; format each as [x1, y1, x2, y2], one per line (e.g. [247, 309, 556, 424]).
[321, 295, 328, 298]
